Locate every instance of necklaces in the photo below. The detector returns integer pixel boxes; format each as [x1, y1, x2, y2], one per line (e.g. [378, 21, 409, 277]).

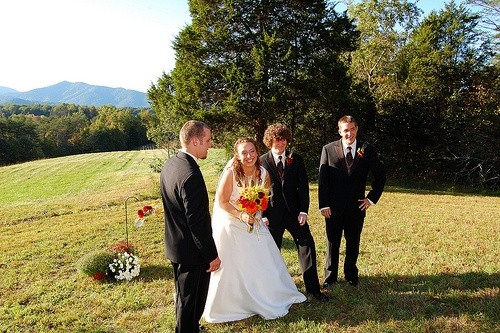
[243, 165, 257, 180]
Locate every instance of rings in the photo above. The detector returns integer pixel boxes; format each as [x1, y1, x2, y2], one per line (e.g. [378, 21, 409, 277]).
[212, 269, 215, 271]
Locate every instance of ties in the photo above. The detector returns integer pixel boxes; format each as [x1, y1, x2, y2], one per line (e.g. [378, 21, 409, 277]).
[346, 147, 353, 166]
[276, 156, 284, 181]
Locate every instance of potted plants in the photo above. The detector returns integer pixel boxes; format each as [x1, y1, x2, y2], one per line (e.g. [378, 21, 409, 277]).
[75, 249, 117, 280]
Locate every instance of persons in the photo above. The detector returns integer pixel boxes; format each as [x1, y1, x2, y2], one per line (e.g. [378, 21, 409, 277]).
[160, 119, 220, 333]
[258, 123, 329, 303]
[318, 116, 389, 288]
[202, 136, 307, 324]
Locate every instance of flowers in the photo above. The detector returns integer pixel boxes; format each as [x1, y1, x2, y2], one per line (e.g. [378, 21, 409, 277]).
[357, 147, 365, 158]
[285, 156, 293, 166]
[233, 186, 271, 233]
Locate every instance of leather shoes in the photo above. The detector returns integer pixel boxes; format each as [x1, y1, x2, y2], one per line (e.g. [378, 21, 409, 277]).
[312, 292, 329, 302]
[348, 279, 359, 286]
[322, 280, 333, 288]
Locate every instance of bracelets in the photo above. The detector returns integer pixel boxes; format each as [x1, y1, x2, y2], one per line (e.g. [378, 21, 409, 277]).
[239, 211, 244, 223]
